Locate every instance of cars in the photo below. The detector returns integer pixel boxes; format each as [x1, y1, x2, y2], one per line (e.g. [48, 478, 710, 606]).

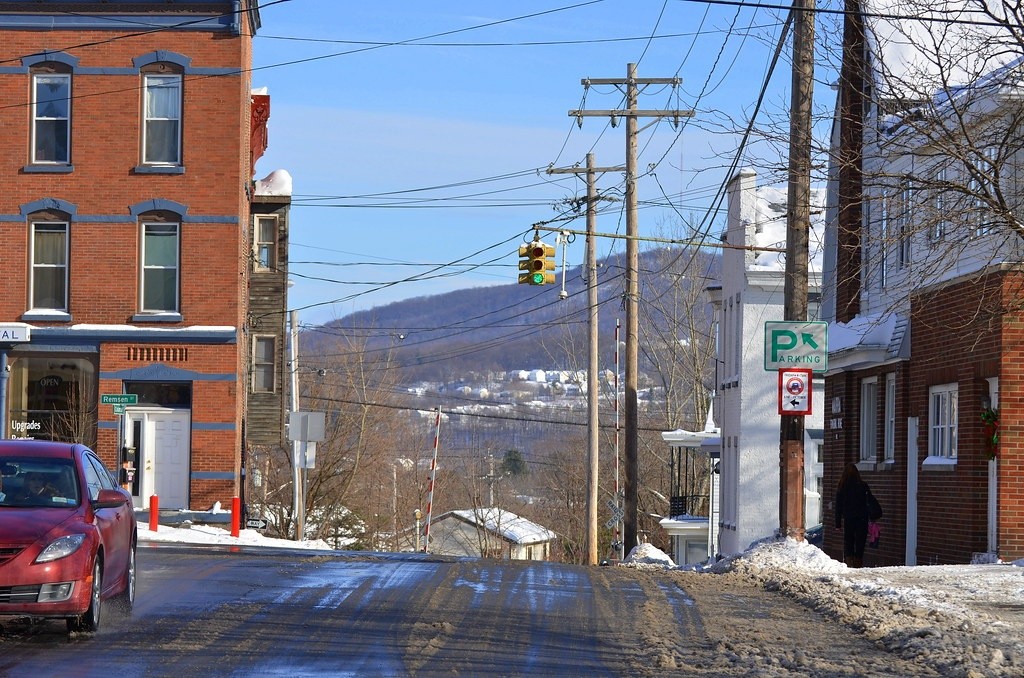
[1, 438, 139, 637]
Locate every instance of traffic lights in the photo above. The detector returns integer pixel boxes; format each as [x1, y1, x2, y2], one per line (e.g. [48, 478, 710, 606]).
[517, 244, 556, 286]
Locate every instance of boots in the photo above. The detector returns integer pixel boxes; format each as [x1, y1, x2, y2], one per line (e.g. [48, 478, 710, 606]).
[846, 555, 862, 567]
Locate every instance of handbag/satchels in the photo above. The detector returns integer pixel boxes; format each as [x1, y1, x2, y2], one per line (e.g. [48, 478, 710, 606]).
[868, 521, 881, 549]
[865, 481, 883, 522]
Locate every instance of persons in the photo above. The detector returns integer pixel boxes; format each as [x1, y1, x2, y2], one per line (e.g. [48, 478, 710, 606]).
[16, 472, 60, 500]
[835, 464, 871, 568]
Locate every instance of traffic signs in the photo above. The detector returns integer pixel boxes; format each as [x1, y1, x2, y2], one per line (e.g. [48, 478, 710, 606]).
[777, 367, 813, 415]
[764, 320, 829, 373]
